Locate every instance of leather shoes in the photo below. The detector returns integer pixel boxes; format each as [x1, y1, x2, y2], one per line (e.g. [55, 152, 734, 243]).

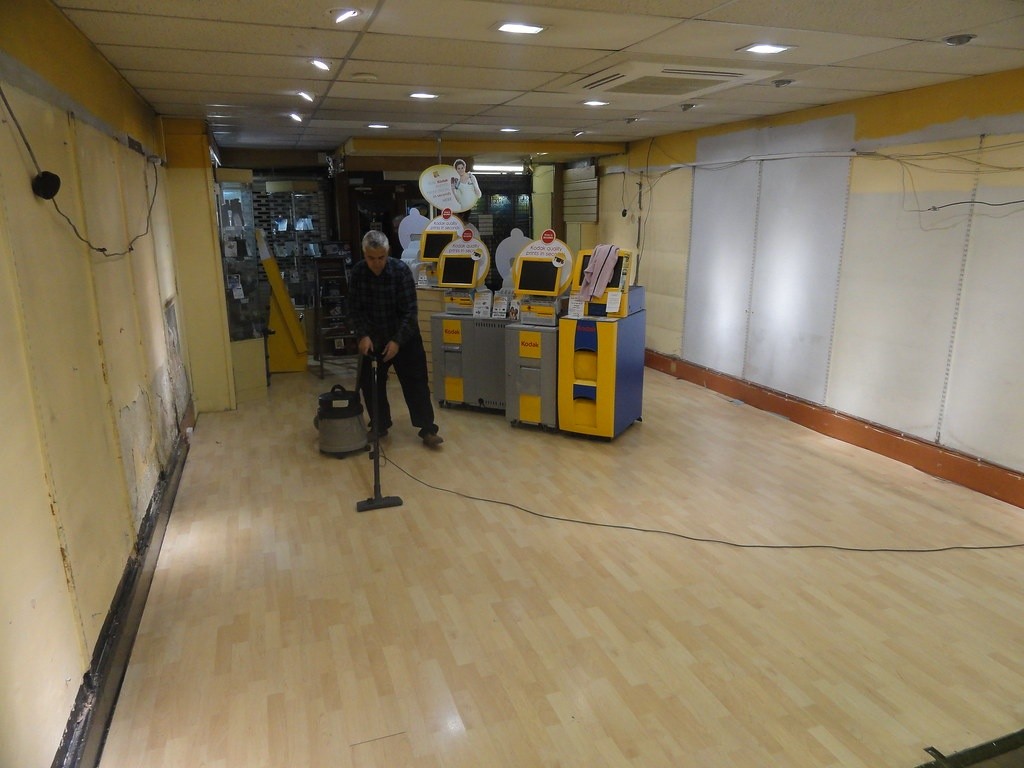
[423, 431, 444, 446]
[363, 426, 388, 444]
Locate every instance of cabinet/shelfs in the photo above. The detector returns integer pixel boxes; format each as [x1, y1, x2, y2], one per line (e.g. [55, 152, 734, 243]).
[214, 168, 325, 389]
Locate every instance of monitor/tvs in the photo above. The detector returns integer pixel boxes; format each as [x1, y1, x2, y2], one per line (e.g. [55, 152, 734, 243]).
[418, 229, 477, 288]
[514, 256, 561, 296]
[571, 249, 632, 294]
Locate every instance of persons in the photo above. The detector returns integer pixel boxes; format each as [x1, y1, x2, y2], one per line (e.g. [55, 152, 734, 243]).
[346, 230, 445, 445]
[451, 159, 482, 210]
